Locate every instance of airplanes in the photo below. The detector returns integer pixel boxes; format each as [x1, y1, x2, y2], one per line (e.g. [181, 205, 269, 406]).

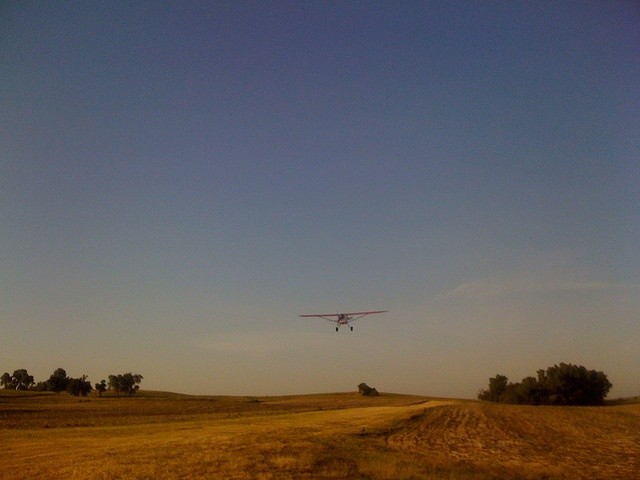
[298, 312, 388, 332]
